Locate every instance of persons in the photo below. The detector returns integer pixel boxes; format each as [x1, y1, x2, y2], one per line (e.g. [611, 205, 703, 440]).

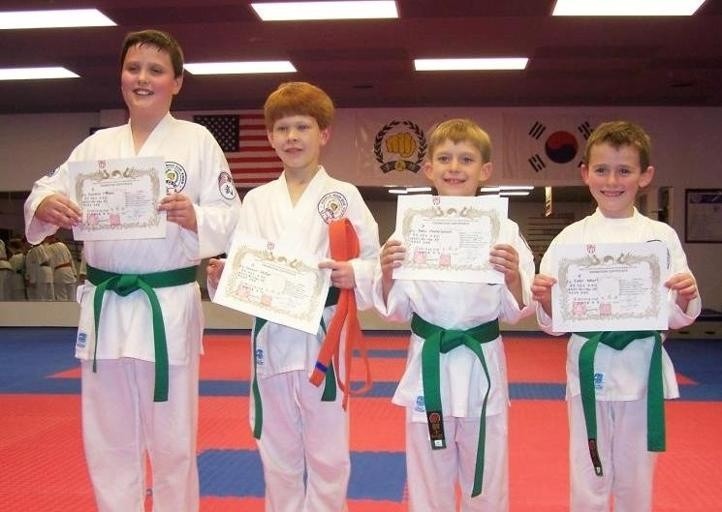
[372, 119, 536, 512]
[0, 234, 88, 302]
[23, 30, 242, 512]
[207, 82, 380, 512]
[530, 122, 702, 512]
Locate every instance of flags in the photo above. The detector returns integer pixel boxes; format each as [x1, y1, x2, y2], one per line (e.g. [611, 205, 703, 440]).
[354, 109, 452, 186]
[193, 113, 284, 189]
[503, 112, 625, 181]
